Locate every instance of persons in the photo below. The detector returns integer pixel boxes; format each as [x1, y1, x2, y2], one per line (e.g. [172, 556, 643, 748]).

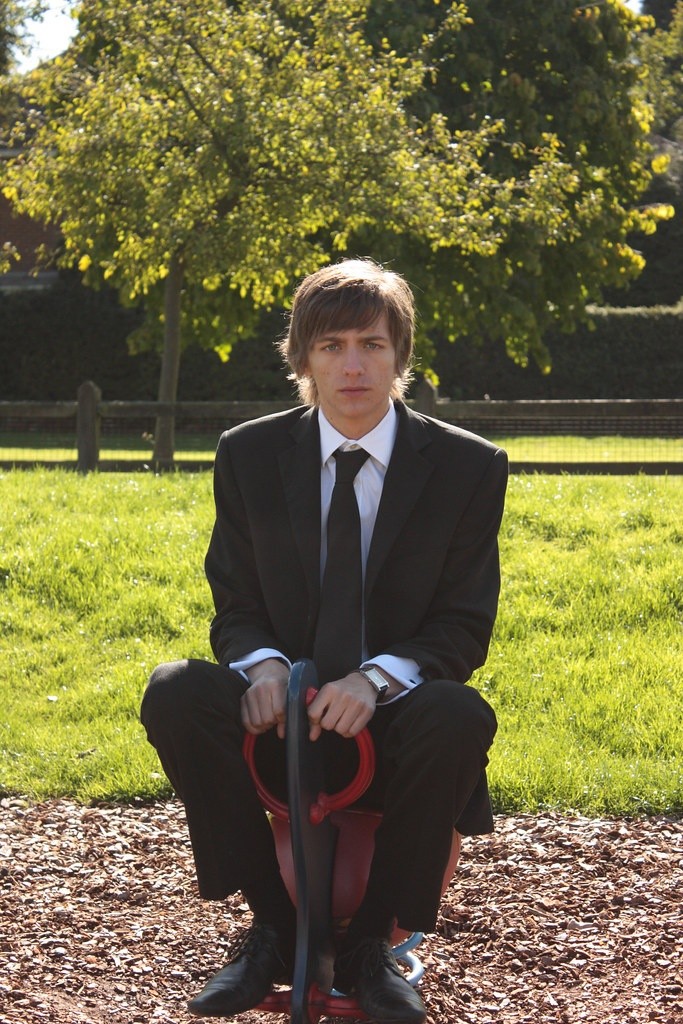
[142, 258, 509, 1024]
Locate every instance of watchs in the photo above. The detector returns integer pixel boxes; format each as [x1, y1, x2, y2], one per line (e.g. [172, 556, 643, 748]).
[347, 667, 390, 703]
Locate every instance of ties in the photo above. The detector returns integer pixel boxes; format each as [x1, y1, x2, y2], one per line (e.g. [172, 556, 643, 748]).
[314, 447, 372, 688]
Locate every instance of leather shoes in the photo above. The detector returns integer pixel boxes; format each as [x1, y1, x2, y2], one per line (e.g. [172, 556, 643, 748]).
[334, 915, 427, 1024]
[188, 910, 297, 1016]
